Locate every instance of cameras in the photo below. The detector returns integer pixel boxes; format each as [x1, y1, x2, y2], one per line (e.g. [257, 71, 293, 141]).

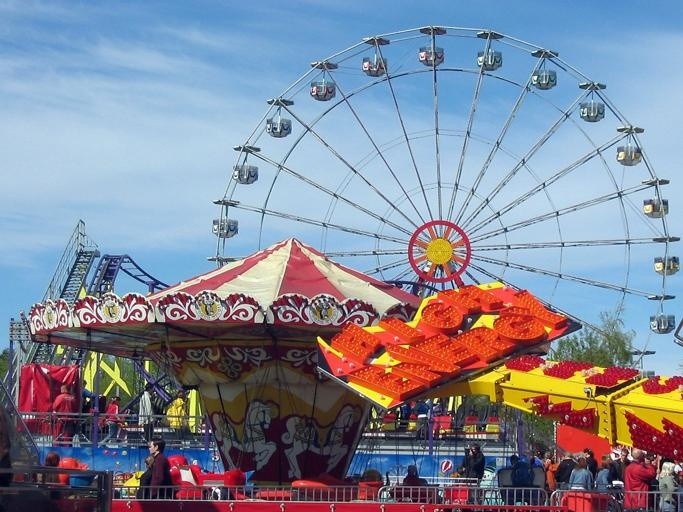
[465, 448, 473, 452]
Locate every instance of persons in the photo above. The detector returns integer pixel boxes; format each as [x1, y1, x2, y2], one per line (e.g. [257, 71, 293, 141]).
[0, 431, 14, 488]
[138, 383, 155, 442]
[414, 398, 429, 439]
[465, 445, 486, 504]
[166, 391, 189, 440]
[147, 438, 176, 499]
[44, 453, 61, 483]
[508, 442, 683, 512]
[136, 455, 154, 500]
[487, 410, 500, 422]
[403, 465, 429, 486]
[52, 384, 79, 447]
[465, 410, 479, 422]
[97, 396, 121, 448]
[82, 380, 97, 400]
